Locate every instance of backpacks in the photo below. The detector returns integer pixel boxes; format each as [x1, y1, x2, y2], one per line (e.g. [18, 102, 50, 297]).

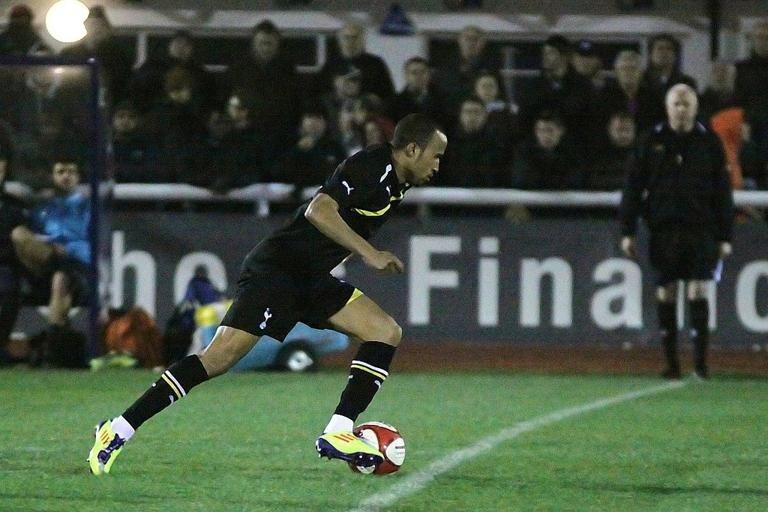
[102, 274, 350, 370]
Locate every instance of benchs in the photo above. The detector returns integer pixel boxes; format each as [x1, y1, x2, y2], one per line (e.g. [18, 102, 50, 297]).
[83, 23, 347, 76]
[418, 26, 653, 108]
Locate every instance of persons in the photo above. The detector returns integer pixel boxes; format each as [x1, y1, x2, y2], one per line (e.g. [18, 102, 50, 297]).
[0, 153, 29, 360]
[618, 82, 734, 383]
[11, 156, 94, 363]
[0, 0, 766, 223]
[87, 113, 448, 476]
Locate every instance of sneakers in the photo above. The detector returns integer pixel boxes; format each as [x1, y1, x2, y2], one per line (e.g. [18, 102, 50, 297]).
[86, 418, 127, 476]
[315, 432, 385, 467]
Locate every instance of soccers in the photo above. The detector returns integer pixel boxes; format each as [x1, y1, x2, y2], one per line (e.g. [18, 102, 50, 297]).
[348, 421, 407, 475]
